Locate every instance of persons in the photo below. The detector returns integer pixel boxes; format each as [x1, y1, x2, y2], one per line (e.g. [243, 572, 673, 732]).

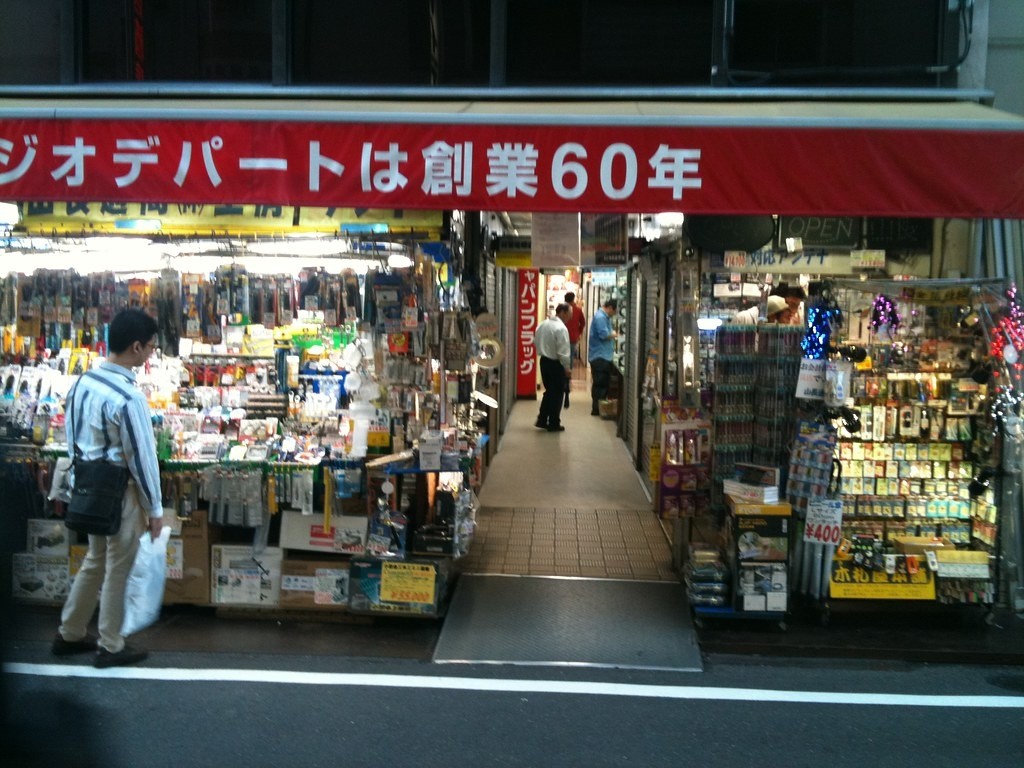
[588, 298, 618, 416]
[731, 284, 805, 327]
[52, 310, 163, 669]
[534, 304, 572, 432]
[564, 292, 585, 369]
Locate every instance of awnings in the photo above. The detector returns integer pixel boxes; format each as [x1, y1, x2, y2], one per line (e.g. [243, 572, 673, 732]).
[0, 88, 1024, 219]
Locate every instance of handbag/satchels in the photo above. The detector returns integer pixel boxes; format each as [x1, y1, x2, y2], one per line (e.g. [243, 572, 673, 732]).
[118, 525, 172, 637]
[65, 459, 130, 535]
[48, 457, 72, 505]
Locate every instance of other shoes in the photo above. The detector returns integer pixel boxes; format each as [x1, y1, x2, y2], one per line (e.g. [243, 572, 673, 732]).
[94, 645, 148, 668]
[564, 396, 569, 408]
[50, 632, 98, 658]
[591, 408, 600, 415]
[535, 419, 549, 429]
[547, 424, 564, 433]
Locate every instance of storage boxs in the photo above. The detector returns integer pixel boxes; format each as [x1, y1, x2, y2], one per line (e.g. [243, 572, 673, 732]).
[725, 494, 792, 563]
[13, 519, 105, 604]
[161, 511, 370, 612]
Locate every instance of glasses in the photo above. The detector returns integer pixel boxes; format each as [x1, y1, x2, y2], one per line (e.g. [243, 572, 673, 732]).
[131, 338, 159, 355]
